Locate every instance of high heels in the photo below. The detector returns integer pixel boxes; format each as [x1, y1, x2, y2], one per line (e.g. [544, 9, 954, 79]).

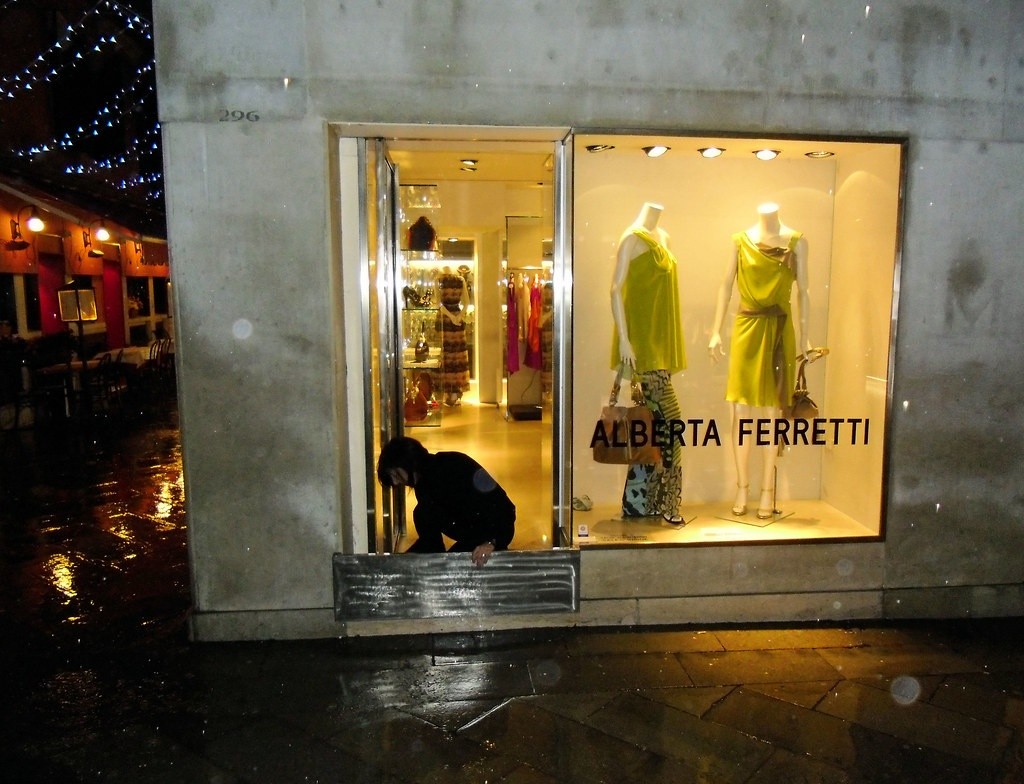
[403, 285, 425, 308]
[732, 483, 748, 515]
[423, 289, 432, 307]
[756, 489, 773, 518]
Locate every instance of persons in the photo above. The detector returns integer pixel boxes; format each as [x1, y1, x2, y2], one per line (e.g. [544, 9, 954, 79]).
[435, 266, 469, 408]
[0, 320, 27, 427]
[609, 202, 686, 526]
[708, 203, 812, 518]
[377, 436, 517, 569]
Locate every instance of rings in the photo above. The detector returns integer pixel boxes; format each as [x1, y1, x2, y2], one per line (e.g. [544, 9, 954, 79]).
[482, 554, 486, 556]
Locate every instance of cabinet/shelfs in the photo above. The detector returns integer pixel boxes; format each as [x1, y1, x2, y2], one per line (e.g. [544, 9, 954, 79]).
[399, 184, 442, 427]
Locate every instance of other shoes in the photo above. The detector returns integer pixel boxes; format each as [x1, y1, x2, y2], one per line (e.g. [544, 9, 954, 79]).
[649, 509, 662, 515]
[662, 512, 683, 524]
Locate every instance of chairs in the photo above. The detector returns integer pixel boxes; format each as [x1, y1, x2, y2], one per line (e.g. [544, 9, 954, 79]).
[10, 336, 171, 464]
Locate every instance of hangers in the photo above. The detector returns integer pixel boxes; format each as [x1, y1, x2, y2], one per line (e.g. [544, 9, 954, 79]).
[508, 266, 546, 288]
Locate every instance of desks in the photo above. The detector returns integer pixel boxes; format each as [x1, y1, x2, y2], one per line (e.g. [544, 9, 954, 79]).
[38, 347, 159, 466]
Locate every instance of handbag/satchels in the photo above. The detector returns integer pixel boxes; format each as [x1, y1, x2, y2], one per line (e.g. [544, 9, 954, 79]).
[593, 361, 673, 469]
[408, 217, 437, 250]
[776, 347, 830, 456]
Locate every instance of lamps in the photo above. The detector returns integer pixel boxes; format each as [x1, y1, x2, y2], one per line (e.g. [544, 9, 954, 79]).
[84, 218, 110, 247]
[10, 204, 46, 240]
